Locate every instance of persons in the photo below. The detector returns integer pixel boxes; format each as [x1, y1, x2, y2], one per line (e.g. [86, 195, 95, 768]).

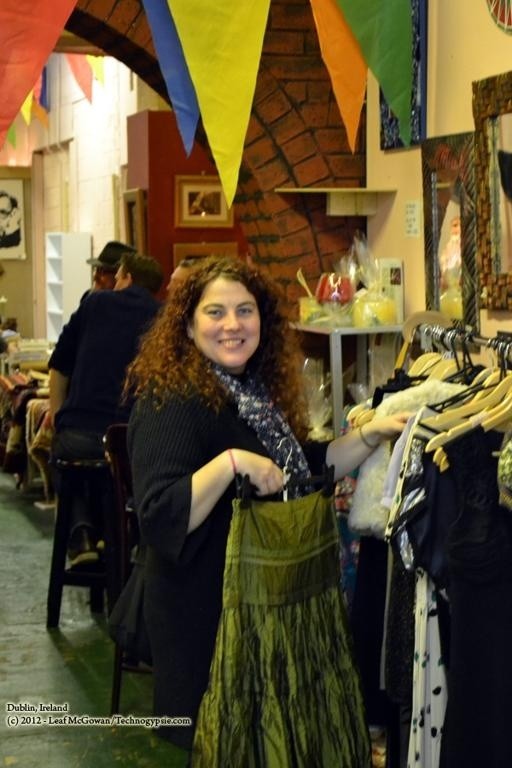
[127, 253, 419, 767]
[47, 255, 166, 574]
[0, 318, 22, 354]
[37, 241, 137, 558]
[0, 190, 24, 250]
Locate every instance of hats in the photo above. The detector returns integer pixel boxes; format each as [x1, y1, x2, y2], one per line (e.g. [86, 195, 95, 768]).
[86, 241, 136, 271]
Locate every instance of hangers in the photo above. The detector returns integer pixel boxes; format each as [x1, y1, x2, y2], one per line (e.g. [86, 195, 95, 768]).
[233, 437, 336, 511]
[392, 318, 512, 472]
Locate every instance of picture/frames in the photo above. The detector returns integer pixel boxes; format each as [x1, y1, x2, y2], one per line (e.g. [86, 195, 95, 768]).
[472, 71, 512, 312]
[174, 242, 236, 268]
[380, 1, 429, 151]
[422, 131, 480, 341]
[175, 175, 236, 229]
[1, 176, 28, 262]
[123, 189, 146, 257]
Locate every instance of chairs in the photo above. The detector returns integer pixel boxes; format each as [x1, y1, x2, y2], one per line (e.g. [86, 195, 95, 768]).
[46, 459, 120, 631]
[111, 422, 166, 720]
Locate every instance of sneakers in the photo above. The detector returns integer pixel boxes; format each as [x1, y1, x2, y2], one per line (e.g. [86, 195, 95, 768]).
[66, 529, 98, 568]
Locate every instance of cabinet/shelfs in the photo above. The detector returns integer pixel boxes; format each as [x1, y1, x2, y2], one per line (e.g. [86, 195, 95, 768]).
[275, 186, 405, 441]
[45, 233, 92, 345]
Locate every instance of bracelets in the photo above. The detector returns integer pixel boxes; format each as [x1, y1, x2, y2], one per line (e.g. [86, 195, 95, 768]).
[228, 450, 237, 476]
[359, 428, 379, 449]
